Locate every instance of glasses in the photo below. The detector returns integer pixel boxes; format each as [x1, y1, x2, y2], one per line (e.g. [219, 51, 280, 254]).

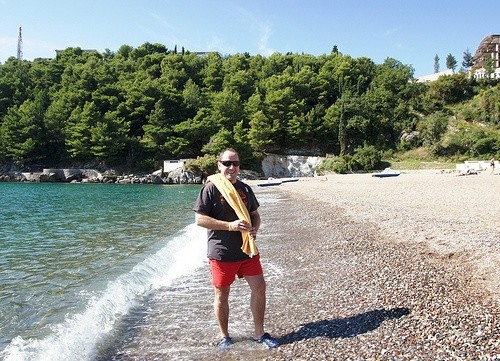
[219, 160, 241, 167]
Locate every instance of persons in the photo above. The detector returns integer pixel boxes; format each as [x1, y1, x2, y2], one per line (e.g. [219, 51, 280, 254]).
[489, 157, 495, 175]
[193, 146, 281, 349]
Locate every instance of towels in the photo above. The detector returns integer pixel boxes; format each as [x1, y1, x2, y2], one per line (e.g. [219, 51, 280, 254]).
[207, 173, 258, 258]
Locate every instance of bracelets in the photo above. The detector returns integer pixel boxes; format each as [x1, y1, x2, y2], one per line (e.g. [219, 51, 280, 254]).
[228, 222, 233, 232]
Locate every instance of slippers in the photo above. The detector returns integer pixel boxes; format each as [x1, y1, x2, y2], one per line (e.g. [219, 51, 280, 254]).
[253, 332, 280, 348]
[218, 337, 233, 348]
